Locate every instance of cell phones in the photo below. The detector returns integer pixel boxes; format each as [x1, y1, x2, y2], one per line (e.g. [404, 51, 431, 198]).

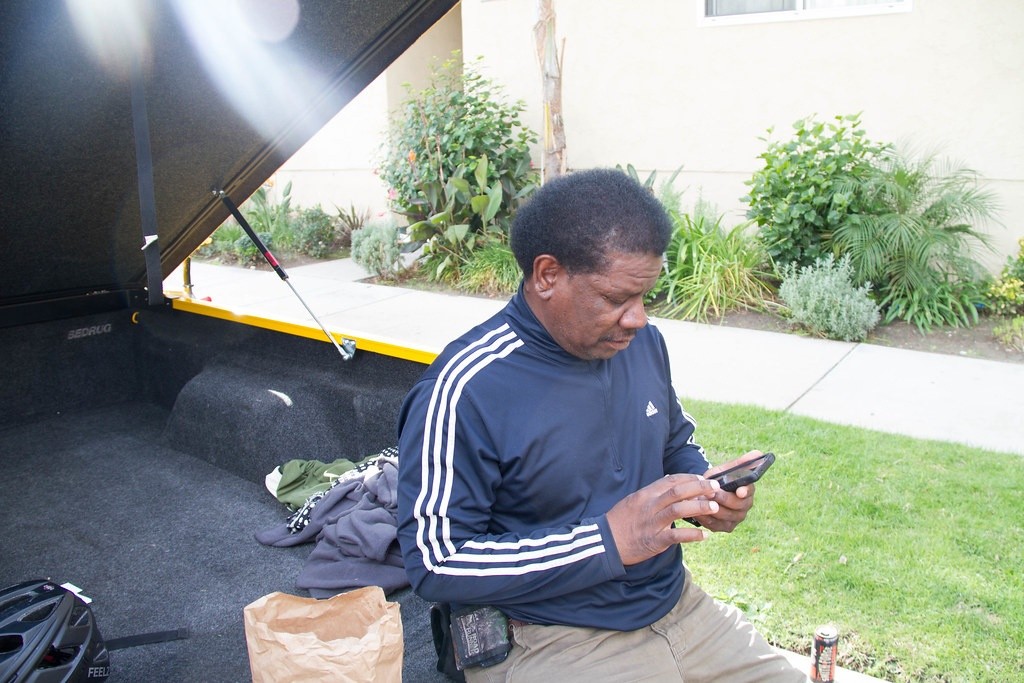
[706, 452, 776, 491]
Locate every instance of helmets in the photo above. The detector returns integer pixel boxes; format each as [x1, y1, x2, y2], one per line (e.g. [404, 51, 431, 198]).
[0, 577, 111, 683]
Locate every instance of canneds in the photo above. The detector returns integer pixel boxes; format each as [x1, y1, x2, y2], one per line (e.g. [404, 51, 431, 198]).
[810, 624, 839, 683]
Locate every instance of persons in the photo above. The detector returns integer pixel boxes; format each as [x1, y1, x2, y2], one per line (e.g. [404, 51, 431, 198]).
[395, 167, 811, 683]
